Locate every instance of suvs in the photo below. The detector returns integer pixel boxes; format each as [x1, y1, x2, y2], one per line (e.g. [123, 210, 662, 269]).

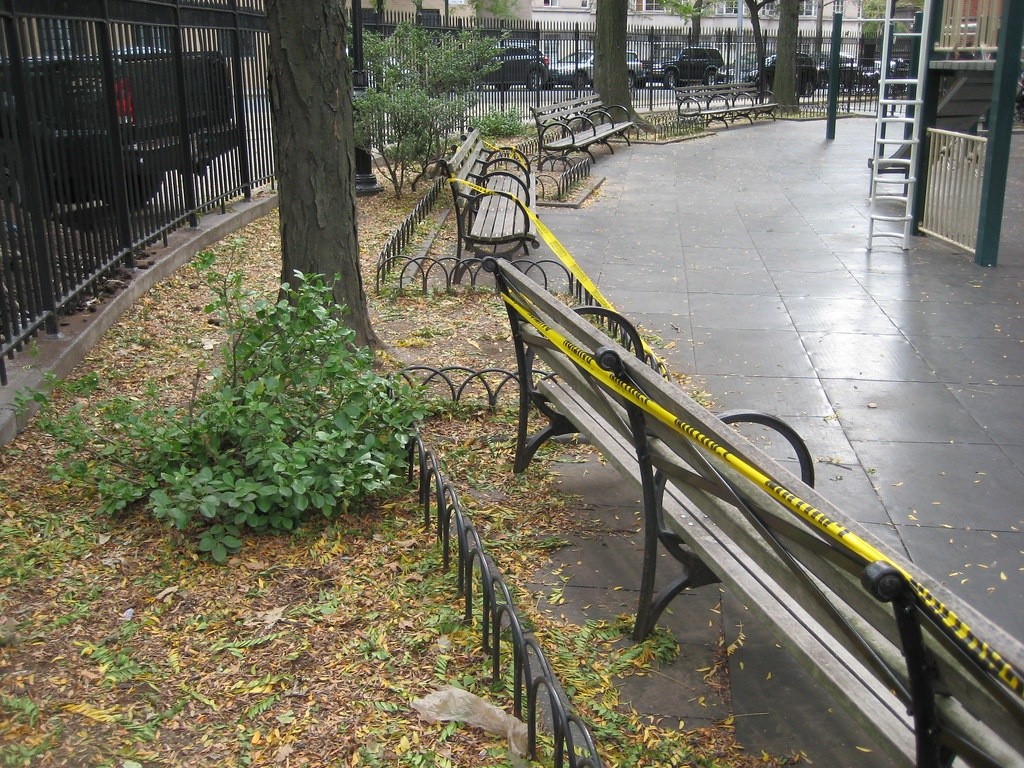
[635, 46, 726, 89]
[543, 48, 644, 90]
[475, 42, 549, 91]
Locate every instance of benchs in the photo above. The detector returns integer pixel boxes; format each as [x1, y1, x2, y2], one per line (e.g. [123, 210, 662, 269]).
[673, 81, 779, 128]
[480, 257, 1024, 768]
[529, 93, 634, 170]
[438, 126, 539, 285]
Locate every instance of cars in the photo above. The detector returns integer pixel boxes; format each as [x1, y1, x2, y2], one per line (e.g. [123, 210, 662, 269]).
[715, 49, 818, 97]
[811, 51, 908, 92]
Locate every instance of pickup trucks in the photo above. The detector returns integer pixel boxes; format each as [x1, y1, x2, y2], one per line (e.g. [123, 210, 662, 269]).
[1, 47, 244, 222]
